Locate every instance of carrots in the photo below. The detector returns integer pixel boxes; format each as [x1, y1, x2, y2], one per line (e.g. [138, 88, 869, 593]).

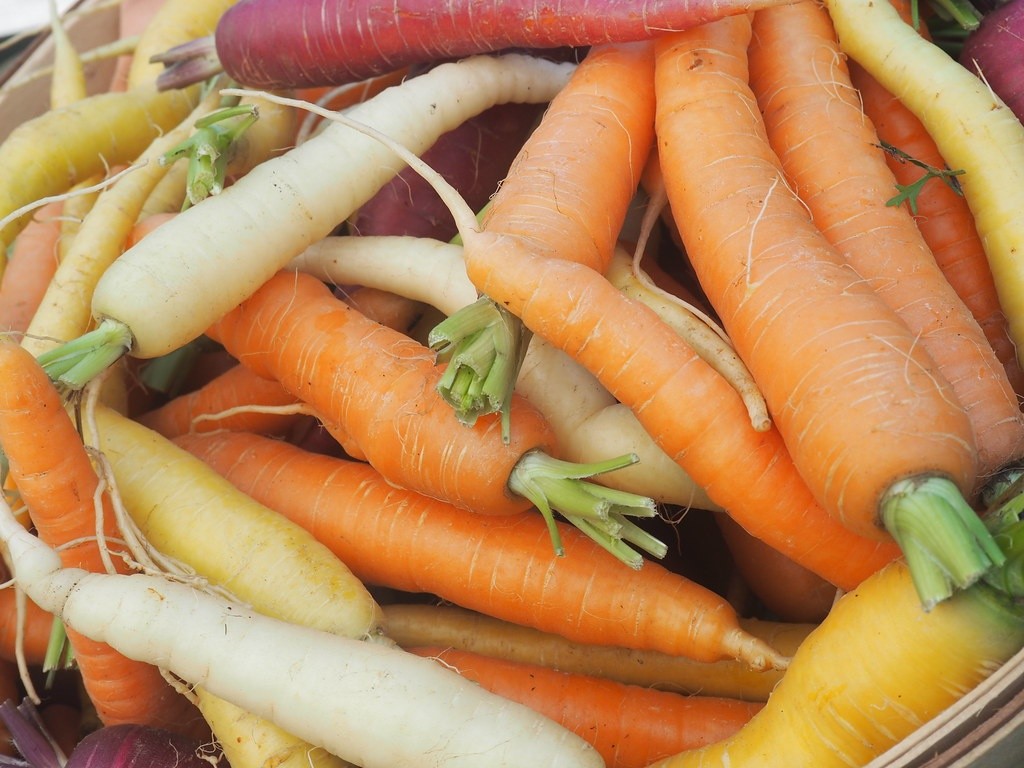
[0, 0, 1024, 768]
